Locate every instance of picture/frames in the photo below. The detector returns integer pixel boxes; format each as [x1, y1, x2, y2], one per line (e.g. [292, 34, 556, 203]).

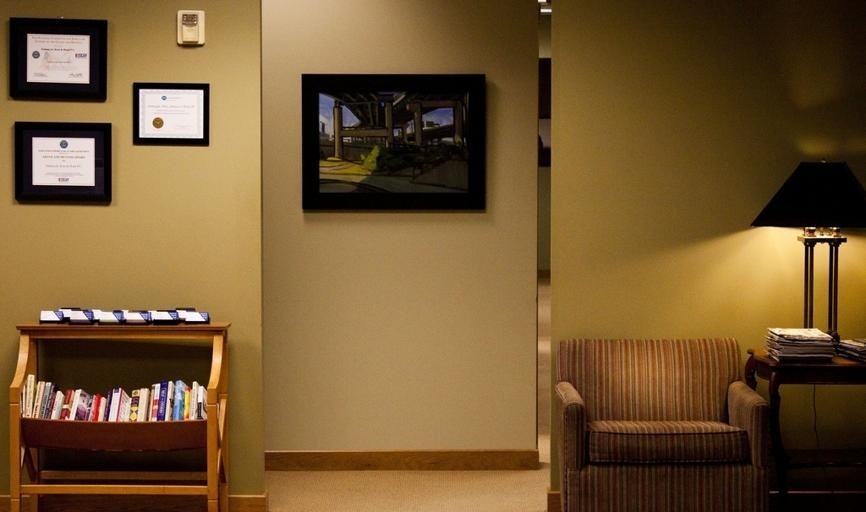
[8, 16, 106, 104]
[12, 122, 113, 207]
[134, 81, 211, 147]
[299, 72, 488, 215]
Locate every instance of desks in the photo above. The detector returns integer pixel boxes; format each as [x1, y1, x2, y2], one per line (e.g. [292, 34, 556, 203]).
[741, 334, 865, 512]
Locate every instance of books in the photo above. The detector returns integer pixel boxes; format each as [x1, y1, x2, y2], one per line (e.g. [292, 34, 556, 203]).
[765, 326, 866, 365]
[21, 373, 207, 422]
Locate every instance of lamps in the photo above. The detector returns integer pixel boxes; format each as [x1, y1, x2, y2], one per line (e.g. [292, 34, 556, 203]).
[750, 149, 866, 345]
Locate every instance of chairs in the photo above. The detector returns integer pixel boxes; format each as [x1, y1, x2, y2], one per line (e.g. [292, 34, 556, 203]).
[552, 337, 777, 511]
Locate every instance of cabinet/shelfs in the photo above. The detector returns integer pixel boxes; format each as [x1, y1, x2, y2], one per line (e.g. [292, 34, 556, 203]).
[10, 310, 237, 511]
